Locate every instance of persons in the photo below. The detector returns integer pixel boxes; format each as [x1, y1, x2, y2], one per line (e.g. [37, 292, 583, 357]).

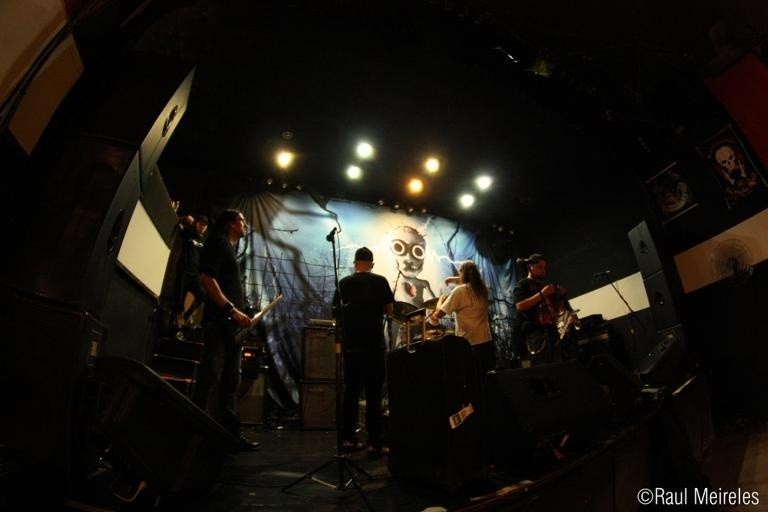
[179, 215, 211, 324]
[200, 208, 260, 451]
[435, 262, 499, 376]
[511, 251, 575, 361]
[331, 247, 394, 458]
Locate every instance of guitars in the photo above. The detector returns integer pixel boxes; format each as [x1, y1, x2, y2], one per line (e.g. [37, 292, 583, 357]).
[213, 295, 281, 355]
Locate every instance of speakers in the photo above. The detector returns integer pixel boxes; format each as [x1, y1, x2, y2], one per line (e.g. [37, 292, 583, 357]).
[627, 219, 677, 279]
[208, 366, 266, 426]
[644, 269, 691, 333]
[302, 382, 344, 431]
[302, 326, 343, 380]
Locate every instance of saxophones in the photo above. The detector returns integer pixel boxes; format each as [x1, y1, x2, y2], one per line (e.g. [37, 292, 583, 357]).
[555, 281, 581, 343]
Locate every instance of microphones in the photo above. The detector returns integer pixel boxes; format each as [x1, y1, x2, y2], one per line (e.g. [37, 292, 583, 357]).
[593, 270, 611, 278]
[516, 258, 536, 265]
[327, 228, 337, 241]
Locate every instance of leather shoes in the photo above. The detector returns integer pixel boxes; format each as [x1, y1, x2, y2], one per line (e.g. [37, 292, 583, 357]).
[366, 446, 389, 460]
[340, 440, 367, 453]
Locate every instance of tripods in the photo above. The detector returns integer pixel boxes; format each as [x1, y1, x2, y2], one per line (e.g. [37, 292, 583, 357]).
[281, 240, 375, 512]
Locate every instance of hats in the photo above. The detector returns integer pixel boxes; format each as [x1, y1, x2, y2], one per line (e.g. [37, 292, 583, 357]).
[354, 247, 373, 261]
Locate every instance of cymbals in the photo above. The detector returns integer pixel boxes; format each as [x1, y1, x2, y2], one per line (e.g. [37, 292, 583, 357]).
[391, 301, 422, 328]
[422, 296, 443, 307]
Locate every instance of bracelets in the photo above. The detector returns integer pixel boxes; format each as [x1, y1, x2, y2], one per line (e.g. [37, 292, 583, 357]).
[223, 302, 234, 312]
